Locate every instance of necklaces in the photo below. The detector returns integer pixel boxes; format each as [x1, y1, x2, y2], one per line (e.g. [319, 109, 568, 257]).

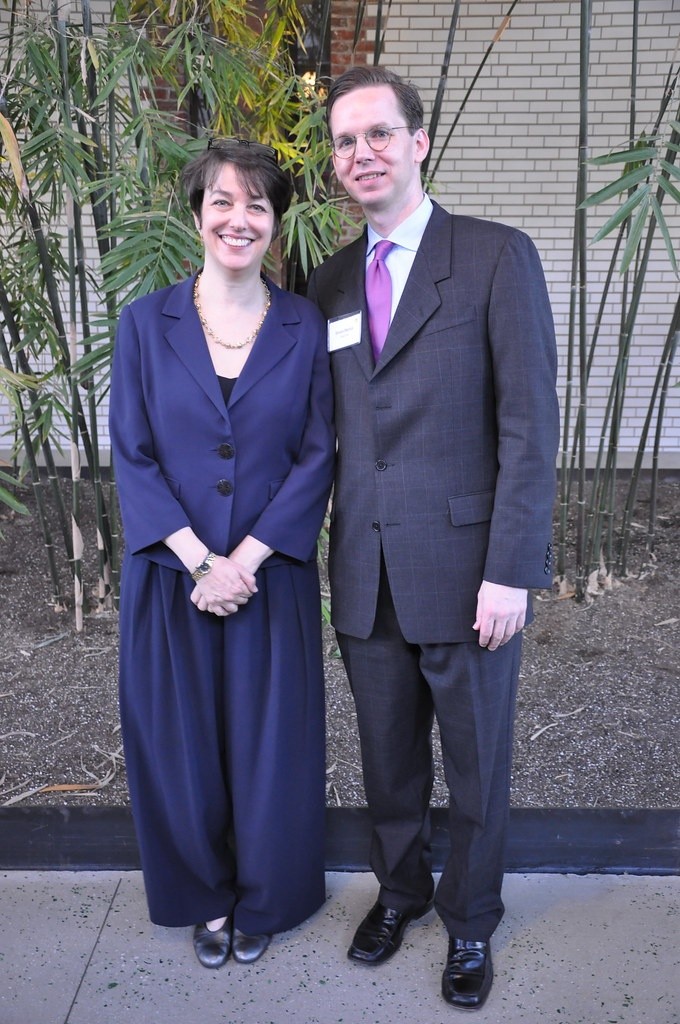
[193, 269, 272, 352]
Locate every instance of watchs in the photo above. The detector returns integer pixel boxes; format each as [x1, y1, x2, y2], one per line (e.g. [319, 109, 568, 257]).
[191, 550, 216, 582]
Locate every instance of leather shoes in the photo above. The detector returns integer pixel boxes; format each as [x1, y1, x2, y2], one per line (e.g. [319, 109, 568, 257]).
[441, 935, 493, 1011]
[347, 882, 434, 966]
[193, 914, 232, 969]
[232, 927, 270, 964]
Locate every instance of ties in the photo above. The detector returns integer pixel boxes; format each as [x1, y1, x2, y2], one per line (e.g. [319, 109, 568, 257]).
[364, 240, 394, 368]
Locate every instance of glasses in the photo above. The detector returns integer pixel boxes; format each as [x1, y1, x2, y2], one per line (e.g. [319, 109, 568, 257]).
[207, 136, 278, 166]
[328, 126, 419, 159]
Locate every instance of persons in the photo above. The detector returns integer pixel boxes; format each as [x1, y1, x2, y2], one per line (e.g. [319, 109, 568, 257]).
[108, 144, 338, 967]
[292, 64, 564, 1014]
